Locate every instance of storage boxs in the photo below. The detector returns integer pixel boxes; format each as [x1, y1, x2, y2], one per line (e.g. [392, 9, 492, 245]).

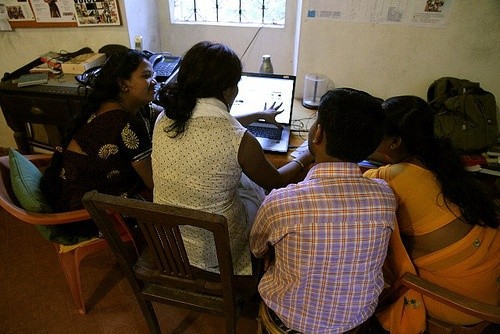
[61, 53, 106, 75]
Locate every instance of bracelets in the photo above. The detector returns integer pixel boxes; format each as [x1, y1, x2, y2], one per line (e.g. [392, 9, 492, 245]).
[293, 159, 303, 167]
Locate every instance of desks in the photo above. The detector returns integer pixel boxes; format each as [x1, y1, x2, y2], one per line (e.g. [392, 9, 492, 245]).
[253, 98, 319, 171]
[357, 136, 500, 226]
[0, 51, 182, 154]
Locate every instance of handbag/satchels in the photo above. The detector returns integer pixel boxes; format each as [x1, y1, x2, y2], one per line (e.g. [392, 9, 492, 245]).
[427, 76, 497, 151]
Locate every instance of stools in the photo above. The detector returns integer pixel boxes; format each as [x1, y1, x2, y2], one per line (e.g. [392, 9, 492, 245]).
[255, 300, 362, 334]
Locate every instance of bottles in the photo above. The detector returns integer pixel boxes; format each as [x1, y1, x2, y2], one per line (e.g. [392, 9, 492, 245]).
[259, 54, 273, 73]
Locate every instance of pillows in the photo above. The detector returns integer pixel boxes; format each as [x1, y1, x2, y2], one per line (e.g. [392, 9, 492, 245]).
[8, 147, 88, 246]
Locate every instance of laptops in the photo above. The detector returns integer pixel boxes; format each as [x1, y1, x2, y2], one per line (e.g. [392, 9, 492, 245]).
[229, 71, 297, 155]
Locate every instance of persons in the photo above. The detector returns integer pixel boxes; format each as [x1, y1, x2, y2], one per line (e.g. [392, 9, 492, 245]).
[250, 87, 396, 334]
[362, 96, 500, 334]
[38, 50, 157, 239]
[151, 40, 315, 275]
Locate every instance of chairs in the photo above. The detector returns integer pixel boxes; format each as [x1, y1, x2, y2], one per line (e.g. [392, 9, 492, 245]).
[81, 191, 265, 334]
[374, 271, 500, 334]
[0, 147, 143, 315]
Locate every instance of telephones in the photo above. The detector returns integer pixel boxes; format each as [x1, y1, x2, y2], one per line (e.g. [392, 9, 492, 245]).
[149, 55, 181, 76]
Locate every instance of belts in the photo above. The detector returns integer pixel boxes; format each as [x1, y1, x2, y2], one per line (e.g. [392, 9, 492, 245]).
[267, 307, 375, 334]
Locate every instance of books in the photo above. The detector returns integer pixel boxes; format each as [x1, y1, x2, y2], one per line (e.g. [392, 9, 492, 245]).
[18, 73, 48, 87]
